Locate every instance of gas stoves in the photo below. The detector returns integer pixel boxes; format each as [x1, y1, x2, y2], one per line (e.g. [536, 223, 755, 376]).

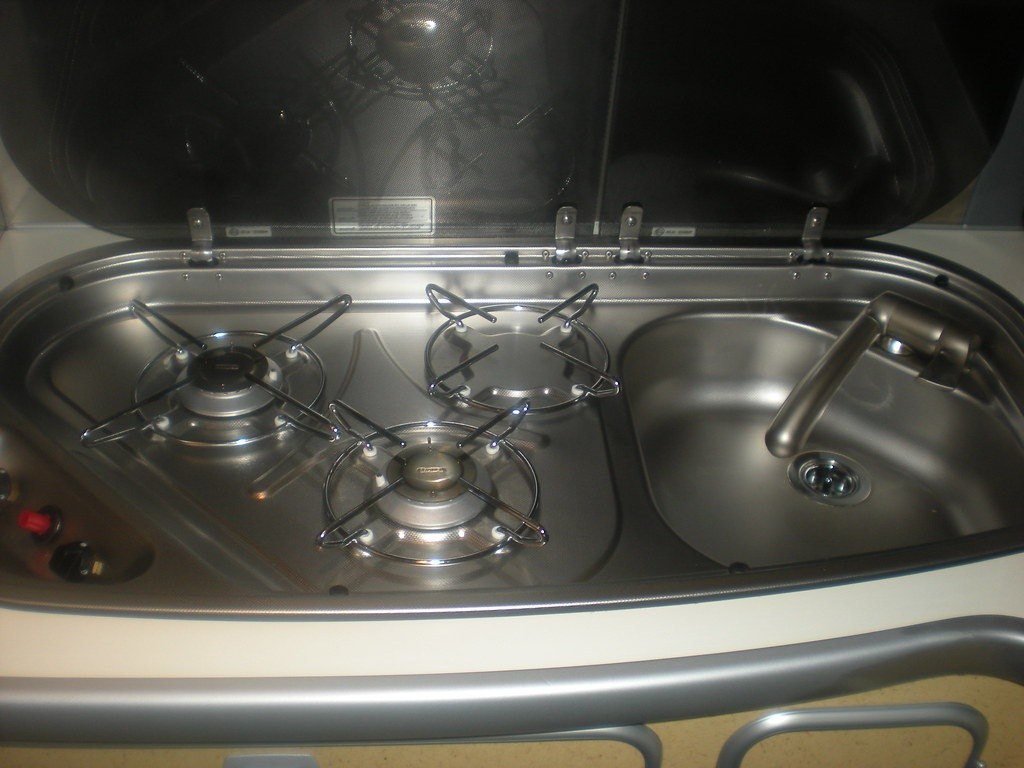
[1, 0, 1023, 241]
[1, 204, 1024, 623]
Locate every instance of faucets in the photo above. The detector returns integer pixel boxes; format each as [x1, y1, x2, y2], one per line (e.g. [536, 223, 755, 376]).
[764, 291, 973, 459]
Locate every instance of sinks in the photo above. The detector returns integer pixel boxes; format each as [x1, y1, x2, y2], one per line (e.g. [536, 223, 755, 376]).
[610, 297, 1024, 577]
